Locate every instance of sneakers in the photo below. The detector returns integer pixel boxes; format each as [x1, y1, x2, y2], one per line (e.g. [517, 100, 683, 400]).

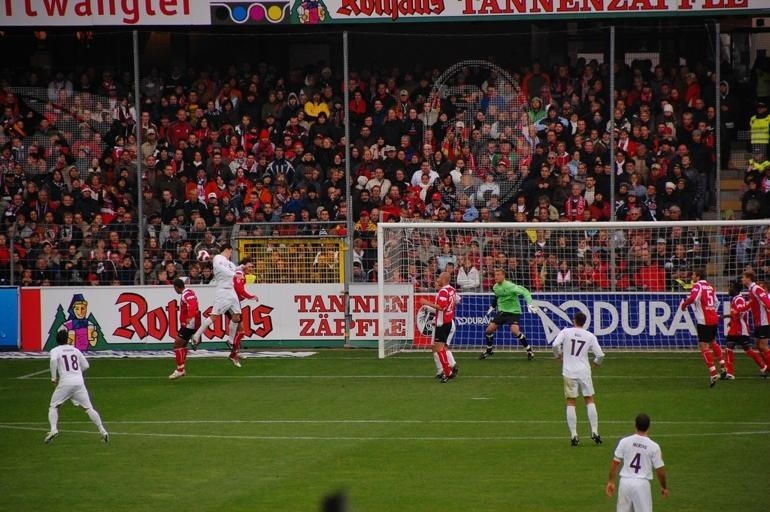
[479, 350, 494, 359]
[226, 339, 234, 349]
[710, 367, 735, 388]
[100, 431, 109, 442]
[591, 433, 602, 444]
[169, 368, 186, 379]
[44, 430, 58, 444]
[570, 436, 579, 447]
[191, 334, 198, 351]
[527, 347, 533, 360]
[434, 364, 459, 383]
[227, 354, 242, 368]
[759, 365, 768, 379]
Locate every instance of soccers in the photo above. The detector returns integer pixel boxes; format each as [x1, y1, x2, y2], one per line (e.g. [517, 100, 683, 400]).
[196, 250, 209, 263]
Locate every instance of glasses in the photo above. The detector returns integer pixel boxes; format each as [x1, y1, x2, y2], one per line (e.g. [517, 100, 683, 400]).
[547, 157, 554, 159]
[586, 179, 592, 181]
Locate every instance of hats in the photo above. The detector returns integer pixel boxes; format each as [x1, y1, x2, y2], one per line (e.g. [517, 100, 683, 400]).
[663, 104, 674, 112]
[260, 129, 269, 138]
[651, 163, 661, 170]
[665, 182, 676, 189]
[208, 192, 217, 199]
[79, 184, 92, 193]
[400, 89, 408, 95]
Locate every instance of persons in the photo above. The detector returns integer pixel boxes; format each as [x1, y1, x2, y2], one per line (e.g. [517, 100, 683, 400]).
[552, 312, 606, 446]
[479, 268, 534, 361]
[167, 279, 198, 379]
[605, 413, 670, 511]
[192, 245, 246, 351]
[0, 55, 769, 291]
[42, 329, 111, 443]
[681, 267, 770, 387]
[420, 272, 457, 384]
[229, 258, 259, 367]
[420, 281, 462, 378]
